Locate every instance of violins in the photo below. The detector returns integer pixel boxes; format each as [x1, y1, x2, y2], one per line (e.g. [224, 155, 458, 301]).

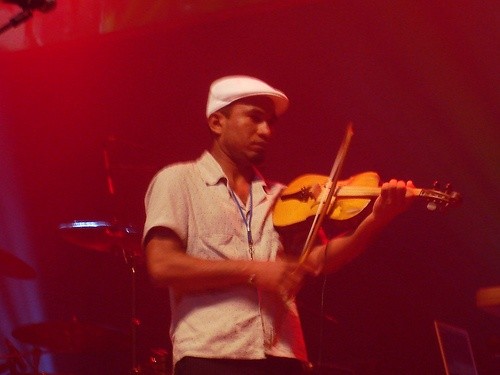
[271, 171, 458, 231]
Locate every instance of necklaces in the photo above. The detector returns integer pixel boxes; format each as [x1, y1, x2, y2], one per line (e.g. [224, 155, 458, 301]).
[227, 174, 253, 243]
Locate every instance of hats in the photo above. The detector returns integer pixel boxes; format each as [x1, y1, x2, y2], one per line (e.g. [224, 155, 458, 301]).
[205, 74, 290, 120]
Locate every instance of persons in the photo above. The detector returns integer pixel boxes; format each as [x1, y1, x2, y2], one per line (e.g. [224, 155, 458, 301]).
[141, 74, 415, 375]
